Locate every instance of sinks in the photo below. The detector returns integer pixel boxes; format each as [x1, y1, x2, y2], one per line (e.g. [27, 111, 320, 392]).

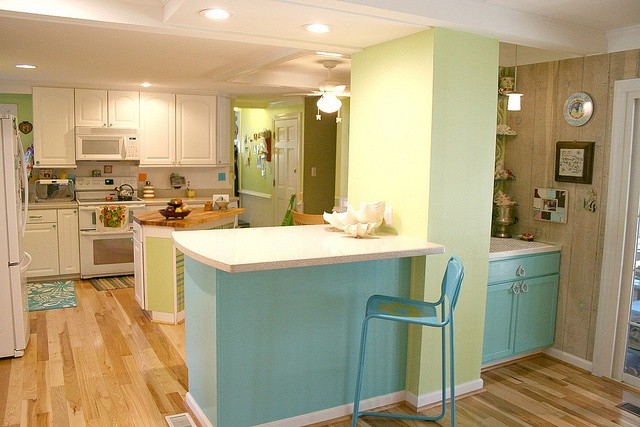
[490, 237, 547, 252]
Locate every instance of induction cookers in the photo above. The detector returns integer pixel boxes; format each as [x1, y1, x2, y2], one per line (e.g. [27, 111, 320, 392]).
[79, 197, 141, 201]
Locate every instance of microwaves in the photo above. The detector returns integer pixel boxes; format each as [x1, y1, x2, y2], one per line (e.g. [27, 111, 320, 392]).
[35, 179, 75, 201]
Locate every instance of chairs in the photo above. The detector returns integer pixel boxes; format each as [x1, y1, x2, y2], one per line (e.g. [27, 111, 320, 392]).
[350, 256, 466, 426]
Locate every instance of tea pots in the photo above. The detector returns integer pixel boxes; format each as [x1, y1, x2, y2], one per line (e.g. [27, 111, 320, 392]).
[115, 183, 135, 200]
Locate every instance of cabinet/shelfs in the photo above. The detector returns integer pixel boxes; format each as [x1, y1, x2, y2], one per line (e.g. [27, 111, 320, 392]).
[75, 88, 140, 129]
[57, 208, 80, 275]
[20, 209, 57, 281]
[216, 95, 233, 167]
[133, 220, 145, 311]
[32, 85, 75, 168]
[141, 94, 216, 168]
[482, 251, 561, 364]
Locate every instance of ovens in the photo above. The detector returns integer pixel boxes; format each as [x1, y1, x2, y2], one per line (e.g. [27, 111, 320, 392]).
[80, 205, 146, 279]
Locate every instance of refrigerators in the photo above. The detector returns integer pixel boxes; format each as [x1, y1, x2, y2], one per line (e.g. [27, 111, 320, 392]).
[1, 112, 32, 359]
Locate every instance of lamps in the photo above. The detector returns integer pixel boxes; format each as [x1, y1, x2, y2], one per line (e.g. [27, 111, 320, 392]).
[316, 60, 344, 124]
[506, 91, 523, 112]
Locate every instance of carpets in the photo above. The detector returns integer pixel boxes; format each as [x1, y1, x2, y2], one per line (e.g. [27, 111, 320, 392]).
[27, 280, 77, 311]
[88, 276, 135, 291]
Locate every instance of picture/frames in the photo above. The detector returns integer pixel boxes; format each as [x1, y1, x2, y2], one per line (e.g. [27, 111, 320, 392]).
[555, 141, 596, 185]
[532, 187, 568, 224]
